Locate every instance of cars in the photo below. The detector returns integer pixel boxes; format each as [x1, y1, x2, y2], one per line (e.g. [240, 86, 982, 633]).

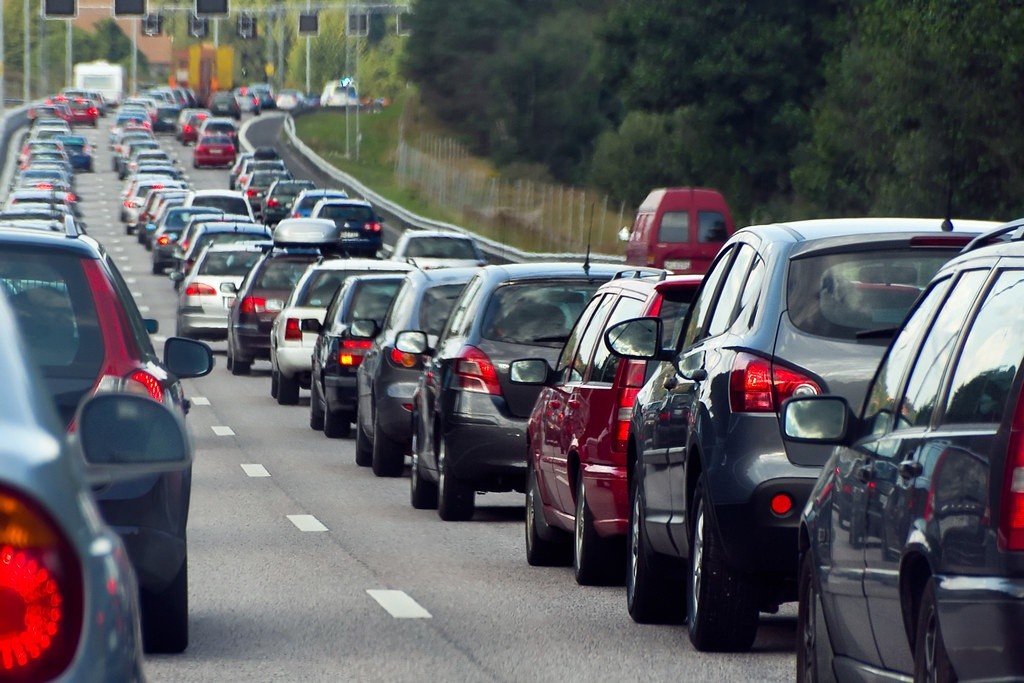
[0, 78, 491, 683]
[391, 261, 678, 524]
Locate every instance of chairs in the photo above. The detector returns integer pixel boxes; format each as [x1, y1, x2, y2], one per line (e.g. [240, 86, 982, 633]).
[12, 287, 79, 365]
[504, 305, 567, 339]
[858, 267, 921, 311]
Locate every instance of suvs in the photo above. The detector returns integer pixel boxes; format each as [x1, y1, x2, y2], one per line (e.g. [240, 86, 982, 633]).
[783, 219, 1024, 683]
[509, 261, 713, 592]
[603, 217, 1019, 655]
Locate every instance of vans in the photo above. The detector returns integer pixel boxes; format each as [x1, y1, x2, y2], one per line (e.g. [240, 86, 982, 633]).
[626, 185, 739, 278]
